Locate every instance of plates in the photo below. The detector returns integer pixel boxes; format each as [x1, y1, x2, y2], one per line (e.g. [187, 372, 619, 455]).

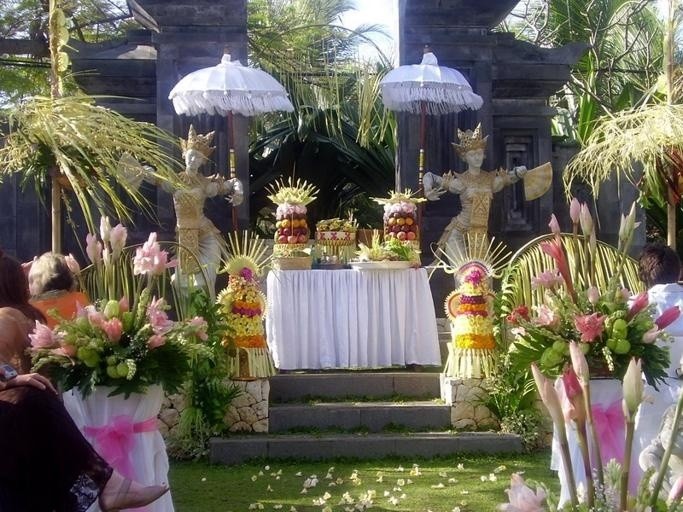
[347, 260, 416, 270]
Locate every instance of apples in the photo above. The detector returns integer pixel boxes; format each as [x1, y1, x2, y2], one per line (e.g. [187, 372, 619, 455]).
[387, 211, 416, 240]
[274, 212, 308, 243]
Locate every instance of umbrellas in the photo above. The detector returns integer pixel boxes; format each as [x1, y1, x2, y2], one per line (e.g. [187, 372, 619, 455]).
[381, 48, 478, 193]
[166, 50, 294, 229]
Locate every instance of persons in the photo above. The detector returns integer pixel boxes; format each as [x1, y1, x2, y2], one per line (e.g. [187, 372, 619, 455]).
[422, 127, 528, 290]
[132, 129, 244, 304]
[618, 242, 682, 444]
[0, 251, 170, 512]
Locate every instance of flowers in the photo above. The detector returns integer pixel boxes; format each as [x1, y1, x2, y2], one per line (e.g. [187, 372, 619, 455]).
[501, 196, 681, 511]
[24, 213, 210, 512]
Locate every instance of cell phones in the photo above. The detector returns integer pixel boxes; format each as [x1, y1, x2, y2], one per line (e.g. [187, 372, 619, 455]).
[0, 365, 17, 381]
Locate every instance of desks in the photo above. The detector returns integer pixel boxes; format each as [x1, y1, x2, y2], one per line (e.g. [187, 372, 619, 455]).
[262, 267, 444, 374]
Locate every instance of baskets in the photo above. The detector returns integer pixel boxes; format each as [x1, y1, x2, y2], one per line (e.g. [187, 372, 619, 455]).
[271, 257, 313, 269]
